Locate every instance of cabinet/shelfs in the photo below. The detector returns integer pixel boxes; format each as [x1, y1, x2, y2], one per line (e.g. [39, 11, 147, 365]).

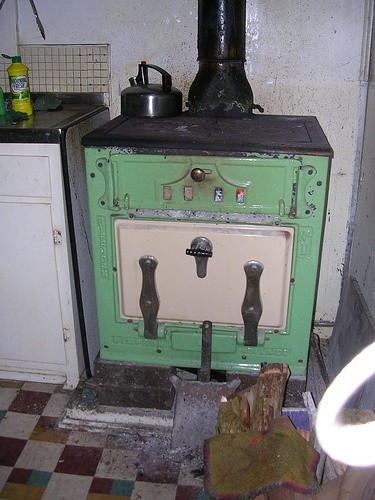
[1, 97, 110, 386]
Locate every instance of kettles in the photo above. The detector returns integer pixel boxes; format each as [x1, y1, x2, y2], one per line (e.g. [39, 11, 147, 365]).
[121, 61, 182, 119]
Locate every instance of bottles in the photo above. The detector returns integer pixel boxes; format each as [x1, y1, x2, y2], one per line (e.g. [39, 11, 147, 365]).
[1, 53, 33, 116]
